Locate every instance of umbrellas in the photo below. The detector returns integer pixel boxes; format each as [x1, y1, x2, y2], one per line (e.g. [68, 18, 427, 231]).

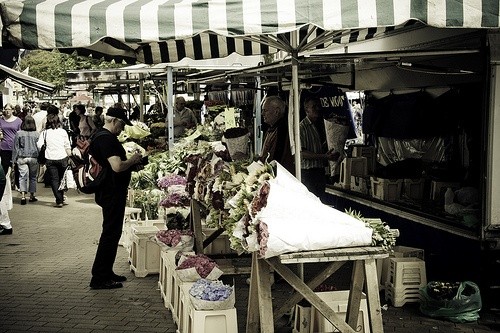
[0, 0, 500, 181]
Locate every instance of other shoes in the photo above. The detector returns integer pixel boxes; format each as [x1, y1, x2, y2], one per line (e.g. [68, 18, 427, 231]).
[62, 201, 69, 205]
[90, 279, 123, 289]
[93, 273, 127, 282]
[0, 228, 12, 234]
[29, 197, 38, 202]
[53, 204, 63, 208]
[21, 197, 27, 203]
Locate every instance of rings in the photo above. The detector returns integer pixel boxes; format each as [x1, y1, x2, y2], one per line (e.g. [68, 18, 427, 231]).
[333, 158, 337, 160]
[332, 156, 336, 158]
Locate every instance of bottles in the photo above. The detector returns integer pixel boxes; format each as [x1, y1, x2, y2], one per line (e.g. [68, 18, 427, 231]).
[444, 187, 454, 213]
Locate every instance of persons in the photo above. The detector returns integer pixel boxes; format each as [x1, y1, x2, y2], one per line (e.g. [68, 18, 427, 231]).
[90, 108, 145, 290]
[165, 97, 197, 139]
[0, 101, 140, 235]
[300, 97, 341, 203]
[148, 95, 168, 123]
[256, 97, 295, 177]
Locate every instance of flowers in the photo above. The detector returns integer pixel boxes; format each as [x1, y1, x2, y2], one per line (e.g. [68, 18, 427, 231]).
[421, 280, 462, 293]
[119, 99, 400, 300]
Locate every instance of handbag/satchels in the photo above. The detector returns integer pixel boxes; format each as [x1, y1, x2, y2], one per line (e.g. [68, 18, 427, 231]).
[420, 280, 482, 324]
[67, 170, 79, 190]
[69, 153, 86, 171]
[38, 129, 47, 164]
[57, 166, 71, 191]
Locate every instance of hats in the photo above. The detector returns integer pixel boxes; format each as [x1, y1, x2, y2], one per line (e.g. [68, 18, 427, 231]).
[106, 105, 133, 127]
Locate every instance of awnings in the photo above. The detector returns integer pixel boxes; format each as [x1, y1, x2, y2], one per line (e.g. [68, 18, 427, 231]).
[0, 64, 57, 96]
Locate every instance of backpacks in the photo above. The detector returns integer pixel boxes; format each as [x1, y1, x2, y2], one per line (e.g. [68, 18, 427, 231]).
[73, 131, 111, 194]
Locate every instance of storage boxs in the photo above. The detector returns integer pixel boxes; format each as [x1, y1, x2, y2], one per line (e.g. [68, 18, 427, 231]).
[379, 246, 423, 290]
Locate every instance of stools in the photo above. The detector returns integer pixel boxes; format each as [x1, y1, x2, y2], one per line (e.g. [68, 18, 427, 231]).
[386, 257, 427, 307]
[123, 218, 237, 333]
[334, 144, 426, 203]
[311, 289, 370, 333]
